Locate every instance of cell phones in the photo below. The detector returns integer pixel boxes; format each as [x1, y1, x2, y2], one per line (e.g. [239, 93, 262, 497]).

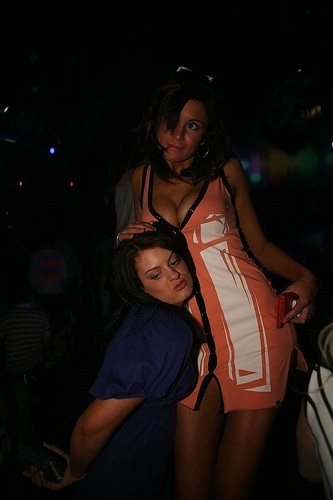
[22, 454, 62, 481]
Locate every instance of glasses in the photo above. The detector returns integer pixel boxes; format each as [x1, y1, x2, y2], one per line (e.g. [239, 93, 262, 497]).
[176, 66, 215, 80]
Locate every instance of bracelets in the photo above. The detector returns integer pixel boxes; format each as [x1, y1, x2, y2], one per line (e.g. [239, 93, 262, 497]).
[115, 232, 120, 244]
[66, 463, 87, 482]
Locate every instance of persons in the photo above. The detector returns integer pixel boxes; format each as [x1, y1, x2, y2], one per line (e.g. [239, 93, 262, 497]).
[295, 325, 333, 499]
[0, 289, 102, 469]
[18, 228, 199, 500]
[113, 70, 319, 500]
